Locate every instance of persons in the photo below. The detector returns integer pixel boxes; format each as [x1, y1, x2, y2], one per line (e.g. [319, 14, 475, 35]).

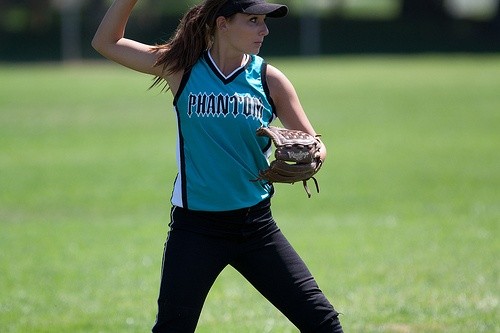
[90, 2, 342, 333]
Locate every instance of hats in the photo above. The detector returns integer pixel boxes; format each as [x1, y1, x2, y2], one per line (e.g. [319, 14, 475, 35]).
[213, 0, 288, 25]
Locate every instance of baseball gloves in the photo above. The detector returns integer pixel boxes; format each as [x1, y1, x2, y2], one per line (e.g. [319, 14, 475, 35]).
[254, 125, 321, 185]
